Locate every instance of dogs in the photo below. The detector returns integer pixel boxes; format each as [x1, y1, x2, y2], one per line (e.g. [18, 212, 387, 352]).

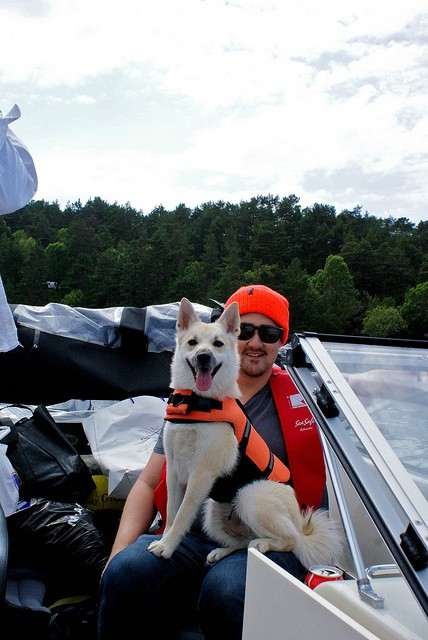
[146, 297, 343, 567]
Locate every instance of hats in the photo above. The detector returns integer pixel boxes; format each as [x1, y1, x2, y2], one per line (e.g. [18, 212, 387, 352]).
[223, 282, 291, 347]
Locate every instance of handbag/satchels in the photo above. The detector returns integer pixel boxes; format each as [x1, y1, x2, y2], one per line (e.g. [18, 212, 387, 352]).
[82, 388, 176, 500]
[0, 401, 96, 505]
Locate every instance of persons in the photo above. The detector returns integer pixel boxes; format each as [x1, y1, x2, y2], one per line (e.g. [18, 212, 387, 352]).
[94, 284, 325, 640]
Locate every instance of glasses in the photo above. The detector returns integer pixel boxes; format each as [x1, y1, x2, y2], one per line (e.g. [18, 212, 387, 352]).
[238, 321, 284, 344]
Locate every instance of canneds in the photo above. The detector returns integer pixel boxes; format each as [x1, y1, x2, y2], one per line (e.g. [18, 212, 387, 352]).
[304, 564, 344, 591]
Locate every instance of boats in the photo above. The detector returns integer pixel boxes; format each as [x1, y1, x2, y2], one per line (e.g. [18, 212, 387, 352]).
[0, 303, 428, 637]
